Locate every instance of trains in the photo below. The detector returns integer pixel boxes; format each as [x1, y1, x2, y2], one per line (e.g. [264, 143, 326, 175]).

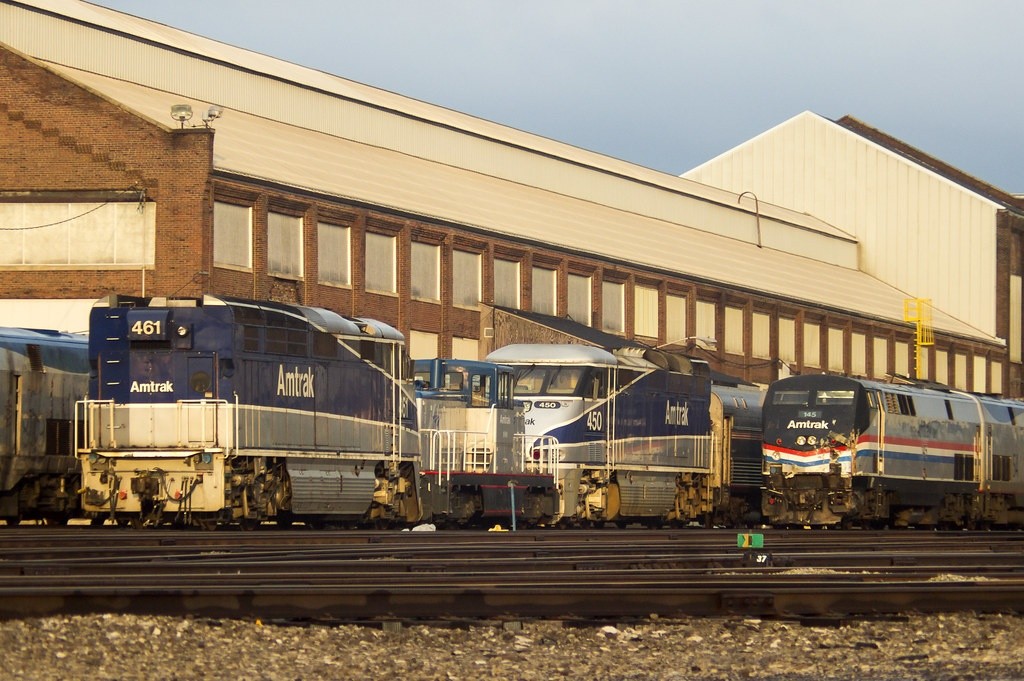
[761, 375, 1024, 530]
[0, 293, 768, 528]
